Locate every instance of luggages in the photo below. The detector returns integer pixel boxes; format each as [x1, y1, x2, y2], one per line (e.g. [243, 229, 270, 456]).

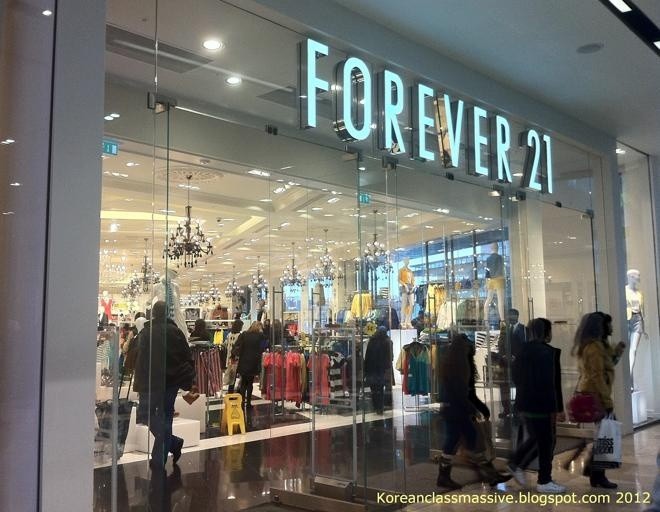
[95, 368, 132, 462]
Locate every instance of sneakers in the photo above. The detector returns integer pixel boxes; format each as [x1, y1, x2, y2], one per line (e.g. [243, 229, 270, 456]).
[172, 437, 183, 464]
[509, 466, 527, 489]
[536, 480, 565, 494]
[498, 407, 513, 417]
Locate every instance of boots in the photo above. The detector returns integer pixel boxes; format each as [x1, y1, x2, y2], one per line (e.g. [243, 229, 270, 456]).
[436, 457, 460, 489]
[486, 463, 511, 487]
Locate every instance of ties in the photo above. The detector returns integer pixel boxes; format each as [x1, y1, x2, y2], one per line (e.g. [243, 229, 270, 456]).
[510, 325, 514, 344]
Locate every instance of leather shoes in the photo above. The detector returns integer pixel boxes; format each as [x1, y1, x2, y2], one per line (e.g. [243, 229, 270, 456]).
[581, 468, 617, 490]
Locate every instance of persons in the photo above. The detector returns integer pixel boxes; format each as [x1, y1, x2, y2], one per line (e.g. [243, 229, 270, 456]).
[146, 263, 191, 343]
[396, 256, 417, 328]
[484, 242, 509, 329]
[622, 267, 651, 393]
[96, 301, 627, 495]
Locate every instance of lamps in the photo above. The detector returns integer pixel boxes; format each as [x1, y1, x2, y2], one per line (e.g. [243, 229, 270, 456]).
[161, 172, 214, 270]
[120, 237, 161, 300]
[184, 209, 395, 308]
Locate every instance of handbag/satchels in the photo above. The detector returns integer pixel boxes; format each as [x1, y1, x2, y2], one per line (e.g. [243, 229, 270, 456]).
[567, 390, 605, 424]
[594, 419, 623, 468]
